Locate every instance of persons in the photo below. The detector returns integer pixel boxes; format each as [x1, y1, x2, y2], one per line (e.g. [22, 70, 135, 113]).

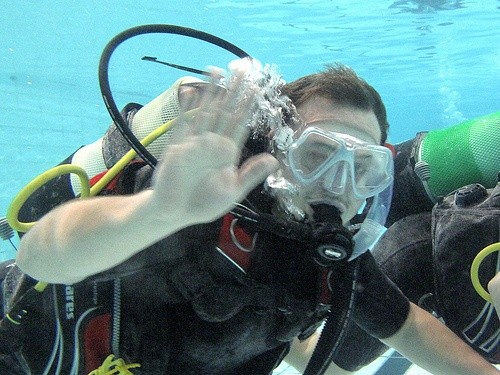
[0, 66, 500, 375]
[281, 173, 500, 375]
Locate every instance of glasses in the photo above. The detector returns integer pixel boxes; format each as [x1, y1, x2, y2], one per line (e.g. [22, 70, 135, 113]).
[287, 126, 397, 200]
